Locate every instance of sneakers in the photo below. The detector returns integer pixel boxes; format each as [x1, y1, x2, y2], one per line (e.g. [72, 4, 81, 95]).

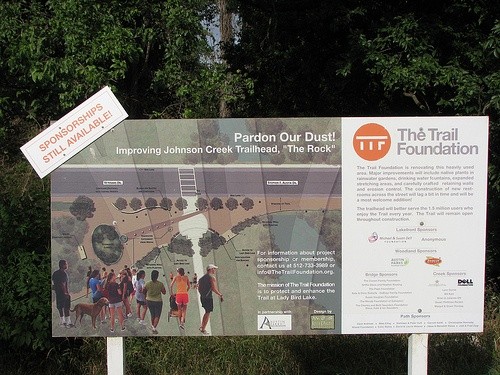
[59, 308, 214, 334]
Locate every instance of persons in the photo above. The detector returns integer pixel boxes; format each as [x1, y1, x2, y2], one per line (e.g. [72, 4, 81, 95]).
[85, 264, 198, 334]
[52, 260, 74, 329]
[197, 264, 223, 334]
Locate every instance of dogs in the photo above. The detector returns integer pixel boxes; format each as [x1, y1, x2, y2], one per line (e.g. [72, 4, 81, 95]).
[70, 297, 110, 328]
